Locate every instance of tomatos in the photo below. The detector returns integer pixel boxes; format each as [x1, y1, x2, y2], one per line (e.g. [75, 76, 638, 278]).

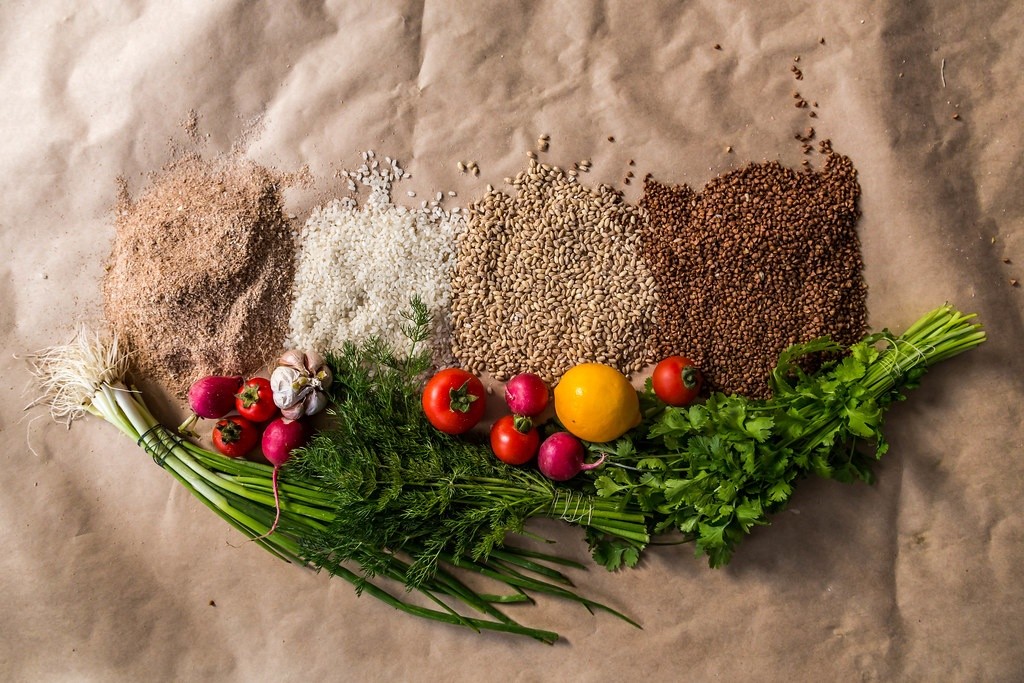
[490, 413, 540, 464]
[422, 368, 484, 434]
[651, 356, 703, 406]
[234, 377, 276, 423]
[212, 415, 257, 456]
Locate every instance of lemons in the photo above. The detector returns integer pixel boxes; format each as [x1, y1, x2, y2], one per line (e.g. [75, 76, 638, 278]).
[554, 364, 641, 441]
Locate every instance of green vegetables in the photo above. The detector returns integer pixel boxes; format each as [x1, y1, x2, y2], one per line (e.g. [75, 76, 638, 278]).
[280, 303, 989, 595]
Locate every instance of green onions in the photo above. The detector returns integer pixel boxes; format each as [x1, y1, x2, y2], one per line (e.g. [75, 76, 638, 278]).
[17, 325, 645, 648]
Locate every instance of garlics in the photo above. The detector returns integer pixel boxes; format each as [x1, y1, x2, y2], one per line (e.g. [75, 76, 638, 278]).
[271, 349, 332, 420]
